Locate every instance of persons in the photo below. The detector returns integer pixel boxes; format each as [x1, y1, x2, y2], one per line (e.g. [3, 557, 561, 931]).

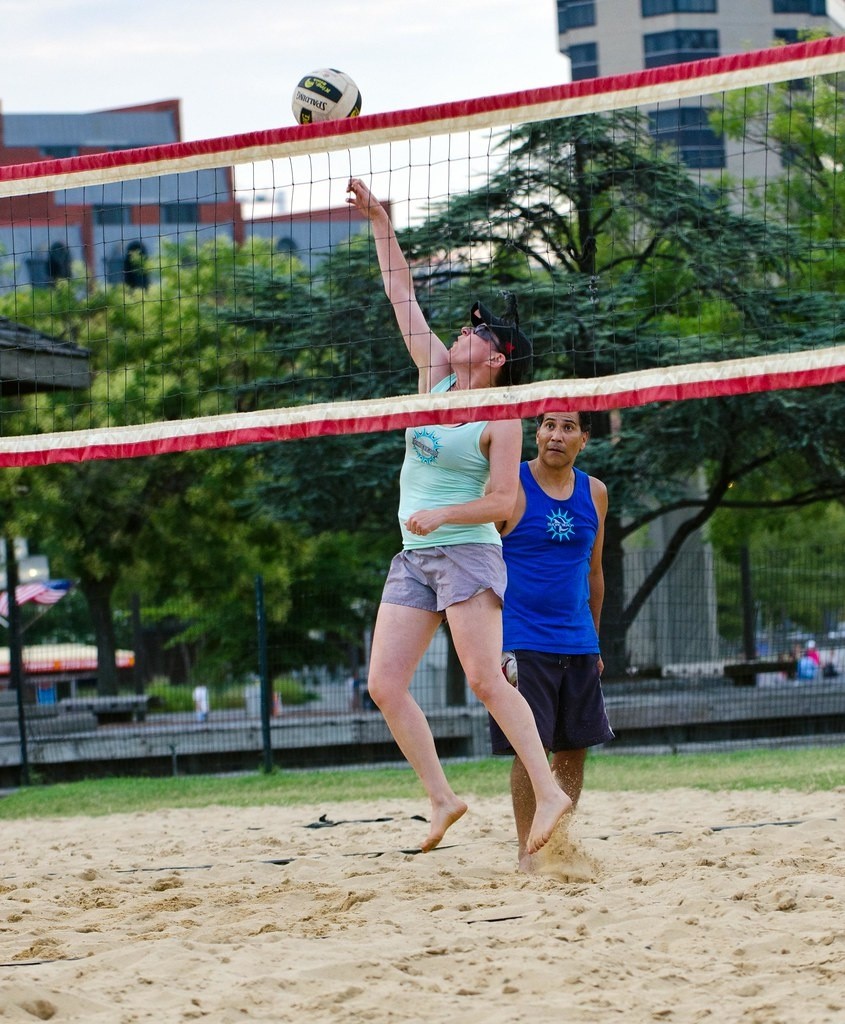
[495, 408, 610, 891]
[191, 683, 211, 722]
[347, 173, 572, 856]
[776, 641, 843, 682]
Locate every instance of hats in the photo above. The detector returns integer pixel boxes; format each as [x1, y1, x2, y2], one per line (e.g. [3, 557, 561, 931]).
[471, 301, 531, 384]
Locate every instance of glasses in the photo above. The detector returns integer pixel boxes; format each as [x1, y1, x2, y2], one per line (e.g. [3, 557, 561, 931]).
[473, 324, 502, 354]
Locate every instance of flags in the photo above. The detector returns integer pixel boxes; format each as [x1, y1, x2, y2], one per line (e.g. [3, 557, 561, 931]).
[0, 578, 71, 618]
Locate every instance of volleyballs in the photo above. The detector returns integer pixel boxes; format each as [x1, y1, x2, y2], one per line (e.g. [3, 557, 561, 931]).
[290, 66, 364, 126]
